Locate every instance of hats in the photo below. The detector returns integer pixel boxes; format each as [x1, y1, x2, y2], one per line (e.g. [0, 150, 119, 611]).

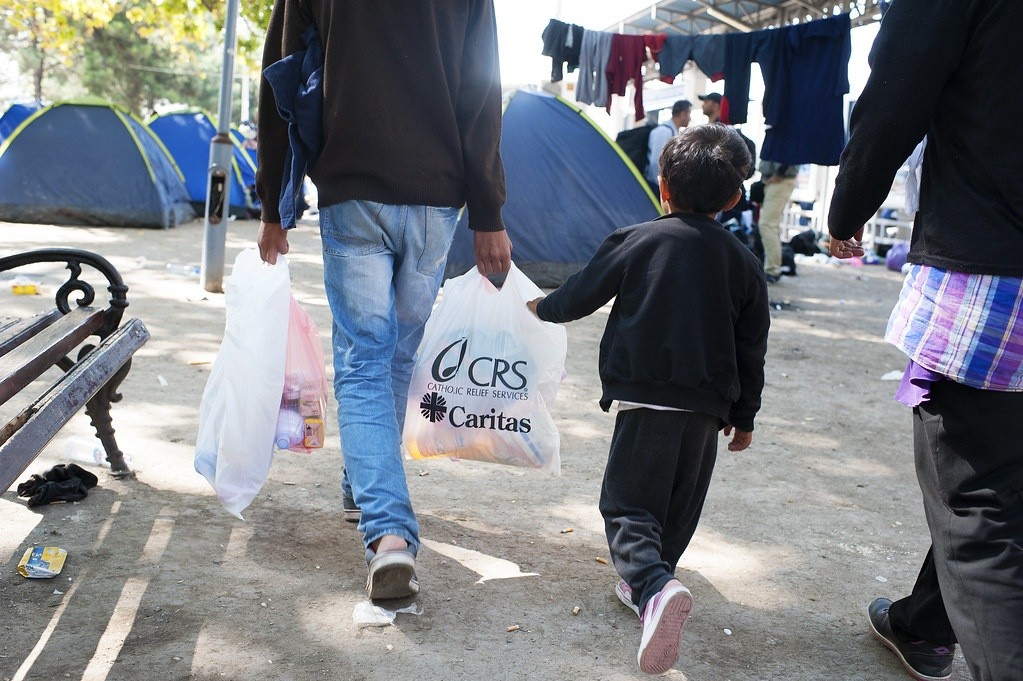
[698, 92, 723, 104]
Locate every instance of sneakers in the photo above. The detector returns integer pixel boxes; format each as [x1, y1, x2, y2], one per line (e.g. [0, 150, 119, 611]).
[636, 579, 693, 675]
[615, 578, 641, 621]
[867, 597, 956, 681]
[342, 494, 363, 521]
[364, 543, 420, 599]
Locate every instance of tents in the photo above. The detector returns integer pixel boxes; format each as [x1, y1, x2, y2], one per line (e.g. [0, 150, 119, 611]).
[0, 98, 195, 229]
[445, 87, 665, 286]
[146, 109, 263, 218]
[0, 102, 44, 144]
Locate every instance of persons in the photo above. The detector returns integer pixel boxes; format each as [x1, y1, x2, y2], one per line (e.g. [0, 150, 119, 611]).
[644, 100, 693, 202]
[698, 93, 723, 122]
[750, 181, 766, 214]
[256, 0, 511, 598]
[828, 0, 1023, 681]
[758, 160, 800, 284]
[525, 123, 770, 675]
[716, 128, 756, 237]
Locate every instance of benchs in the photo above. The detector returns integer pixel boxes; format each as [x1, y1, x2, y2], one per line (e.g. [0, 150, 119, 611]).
[0, 245, 154, 497]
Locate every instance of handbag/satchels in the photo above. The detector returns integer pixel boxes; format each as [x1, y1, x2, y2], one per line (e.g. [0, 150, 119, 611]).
[273, 285, 328, 454]
[401, 253, 567, 478]
[194, 248, 289, 519]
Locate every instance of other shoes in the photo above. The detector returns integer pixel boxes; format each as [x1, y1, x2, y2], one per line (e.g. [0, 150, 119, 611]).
[764, 273, 779, 282]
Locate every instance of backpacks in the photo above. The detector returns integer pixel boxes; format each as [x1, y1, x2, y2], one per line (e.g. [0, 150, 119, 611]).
[616, 123, 674, 177]
[736, 129, 756, 180]
